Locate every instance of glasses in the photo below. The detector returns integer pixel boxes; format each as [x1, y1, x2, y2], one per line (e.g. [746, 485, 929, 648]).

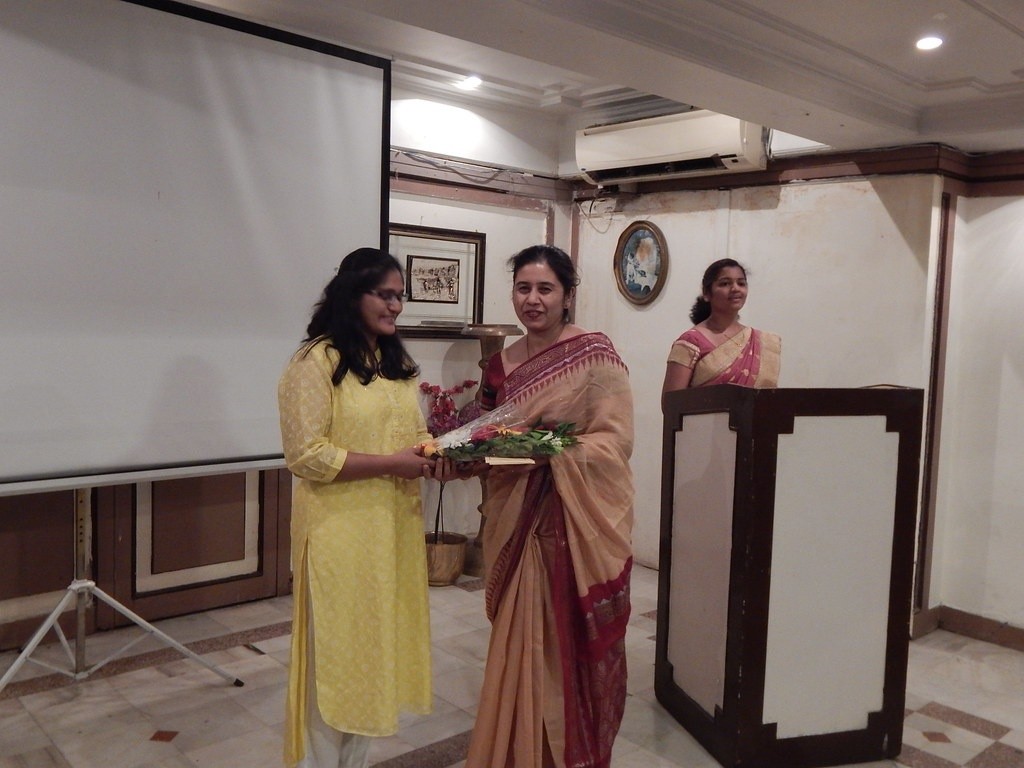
[366, 289, 409, 304]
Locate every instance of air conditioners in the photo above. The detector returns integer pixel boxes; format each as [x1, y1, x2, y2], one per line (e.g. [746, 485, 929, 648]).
[574, 107, 776, 189]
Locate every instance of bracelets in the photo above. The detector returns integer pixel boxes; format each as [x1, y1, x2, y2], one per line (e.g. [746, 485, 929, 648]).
[458, 462, 474, 481]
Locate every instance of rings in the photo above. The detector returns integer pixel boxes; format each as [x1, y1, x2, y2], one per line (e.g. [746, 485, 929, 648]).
[413, 446, 418, 450]
[509, 470, 515, 472]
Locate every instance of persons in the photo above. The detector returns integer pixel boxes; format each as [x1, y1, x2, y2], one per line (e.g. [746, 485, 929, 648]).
[277, 248, 437, 768]
[661, 258, 782, 415]
[422, 244, 636, 768]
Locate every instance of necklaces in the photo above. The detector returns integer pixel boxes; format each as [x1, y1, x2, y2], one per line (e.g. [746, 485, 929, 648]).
[526, 323, 565, 359]
[705, 319, 744, 347]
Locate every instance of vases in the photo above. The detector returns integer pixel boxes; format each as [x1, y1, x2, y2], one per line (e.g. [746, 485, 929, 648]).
[423, 529, 468, 587]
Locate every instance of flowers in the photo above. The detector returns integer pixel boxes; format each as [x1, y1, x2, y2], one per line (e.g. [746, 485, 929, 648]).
[420, 380, 587, 463]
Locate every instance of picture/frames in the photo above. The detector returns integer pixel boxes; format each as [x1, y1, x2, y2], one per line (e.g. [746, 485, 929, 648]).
[130, 469, 266, 602]
[613, 220, 669, 306]
[386, 222, 486, 340]
